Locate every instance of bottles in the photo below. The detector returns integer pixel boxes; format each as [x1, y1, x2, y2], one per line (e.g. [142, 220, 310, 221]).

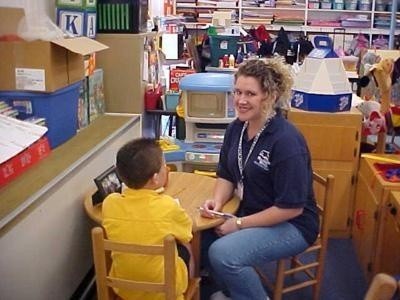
[218, 54, 235, 69]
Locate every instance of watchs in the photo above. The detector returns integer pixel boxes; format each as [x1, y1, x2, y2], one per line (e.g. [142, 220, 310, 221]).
[237, 217, 243, 230]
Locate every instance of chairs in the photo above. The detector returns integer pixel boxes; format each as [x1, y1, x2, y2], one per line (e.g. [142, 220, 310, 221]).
[255, 172, 336, 300]
[90, 227, 202, 299]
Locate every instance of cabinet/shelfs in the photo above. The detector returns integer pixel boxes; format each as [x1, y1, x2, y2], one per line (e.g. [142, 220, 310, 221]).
[281, 105, 363, 241]
[372, 0, 400, 48]
[241, 0, 308, 30]
[308, 0, 374, 47]
[352, 148, 400, 288]
[173, 0, 241, 23]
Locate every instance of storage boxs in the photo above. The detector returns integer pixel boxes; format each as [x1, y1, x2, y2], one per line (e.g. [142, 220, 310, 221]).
[210, 33, 239, 67]
[1, 81, 83, 149]
[0, 34, 112, 93]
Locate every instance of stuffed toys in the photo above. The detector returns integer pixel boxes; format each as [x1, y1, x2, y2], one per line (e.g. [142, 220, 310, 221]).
[350, 50, 400, 154]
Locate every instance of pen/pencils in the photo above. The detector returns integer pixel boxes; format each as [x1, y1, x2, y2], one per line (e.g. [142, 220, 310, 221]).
[196, 207, 238, 219]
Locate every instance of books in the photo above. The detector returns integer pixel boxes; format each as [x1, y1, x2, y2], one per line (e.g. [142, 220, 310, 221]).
[176, 0, 400, 30]
[78, 68, 107, 132]
[0, 101, 45, 128]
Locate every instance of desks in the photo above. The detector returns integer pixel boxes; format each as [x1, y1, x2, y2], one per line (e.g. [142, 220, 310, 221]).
[83, 170, 242, 280]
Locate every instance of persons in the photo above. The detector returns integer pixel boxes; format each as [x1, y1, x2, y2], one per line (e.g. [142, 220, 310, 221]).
[199, 59, 321, 300]
[101, 136, 195, 300]
